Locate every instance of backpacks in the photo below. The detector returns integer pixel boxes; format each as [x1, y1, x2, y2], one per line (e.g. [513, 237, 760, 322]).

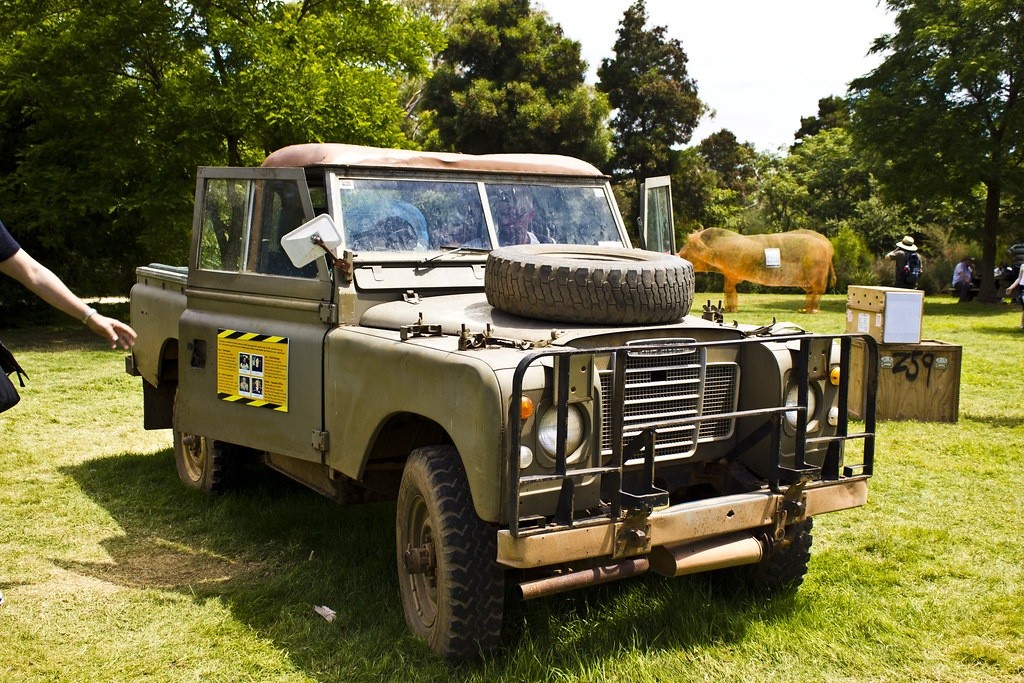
[901, 253, 921, 286]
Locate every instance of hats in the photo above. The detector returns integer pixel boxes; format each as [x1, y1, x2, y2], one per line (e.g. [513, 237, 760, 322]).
[896, 237, 919, 251]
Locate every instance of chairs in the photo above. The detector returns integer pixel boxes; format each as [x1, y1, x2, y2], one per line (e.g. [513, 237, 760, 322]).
[271, 207, 504, 276]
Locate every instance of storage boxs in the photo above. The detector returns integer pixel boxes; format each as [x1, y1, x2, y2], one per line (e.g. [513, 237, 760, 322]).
[847, 285, 924, 343]
[848, 339, 962, 423]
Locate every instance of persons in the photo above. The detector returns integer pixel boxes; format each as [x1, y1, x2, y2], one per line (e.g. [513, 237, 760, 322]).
[0, 217, 137, 414]
[884, 234, 1023, 332]
[465, 188, 556, 251]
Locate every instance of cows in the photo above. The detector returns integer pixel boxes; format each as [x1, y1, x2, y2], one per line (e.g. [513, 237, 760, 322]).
[676, 222, 838, 315]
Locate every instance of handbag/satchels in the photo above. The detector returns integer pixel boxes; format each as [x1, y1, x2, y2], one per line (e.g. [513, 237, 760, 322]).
[0, 340, 29, 414]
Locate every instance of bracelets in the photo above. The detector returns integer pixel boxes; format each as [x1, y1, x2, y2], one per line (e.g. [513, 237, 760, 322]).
[82, 305, 96, 324]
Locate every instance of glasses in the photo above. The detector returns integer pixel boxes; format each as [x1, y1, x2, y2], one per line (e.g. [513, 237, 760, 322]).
[494, 209, 527, 221]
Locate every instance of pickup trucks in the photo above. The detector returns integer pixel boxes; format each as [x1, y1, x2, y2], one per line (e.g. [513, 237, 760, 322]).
[120, 141, 881, 664]
